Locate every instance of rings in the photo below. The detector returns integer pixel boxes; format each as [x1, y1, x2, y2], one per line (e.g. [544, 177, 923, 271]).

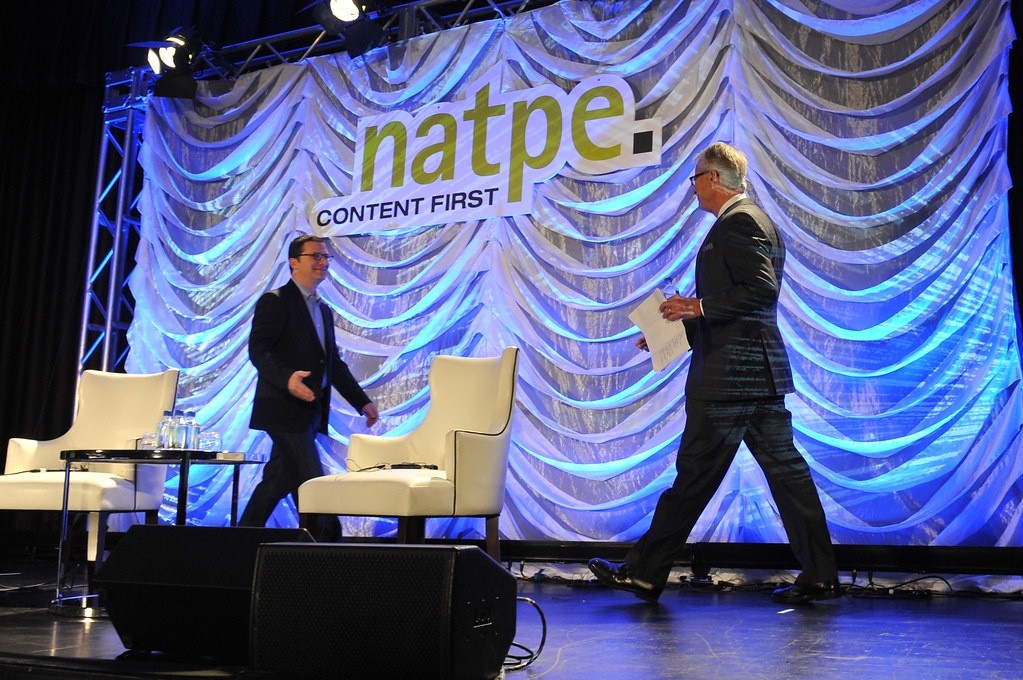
[668, 308, 672, 314]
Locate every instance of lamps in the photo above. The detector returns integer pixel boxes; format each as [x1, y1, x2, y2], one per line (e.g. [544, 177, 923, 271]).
[148, 25, 216, 75]
[330, 0, 385, 23]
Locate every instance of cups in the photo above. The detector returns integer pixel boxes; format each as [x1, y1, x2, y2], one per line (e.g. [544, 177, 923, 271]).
[141, 431, 161, 449]
[198, 431, 222, 451]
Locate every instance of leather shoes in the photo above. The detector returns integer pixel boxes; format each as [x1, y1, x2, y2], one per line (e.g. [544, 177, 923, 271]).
[588, 558, 667, 603]
[772, 577, 842, 602]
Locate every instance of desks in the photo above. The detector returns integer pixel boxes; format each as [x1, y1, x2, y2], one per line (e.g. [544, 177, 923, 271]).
[50, 450, 265, 606]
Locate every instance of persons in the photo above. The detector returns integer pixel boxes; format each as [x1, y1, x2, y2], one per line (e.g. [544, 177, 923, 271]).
[238, 235, 379, 531]
[588, 141, 844, 603]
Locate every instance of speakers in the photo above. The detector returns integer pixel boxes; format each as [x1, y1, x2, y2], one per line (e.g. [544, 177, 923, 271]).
[92, 524, 518, 680]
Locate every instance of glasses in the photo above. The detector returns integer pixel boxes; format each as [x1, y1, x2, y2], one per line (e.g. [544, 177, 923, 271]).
[295, 252, 334, 262]
[690, 171, 719, 185]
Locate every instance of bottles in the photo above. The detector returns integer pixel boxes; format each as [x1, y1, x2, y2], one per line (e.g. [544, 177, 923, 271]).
[185, 411, 200, 449]
[170, 409, 188, 448]
[157, 411, 174, 448]
[663, 277, 683, 322]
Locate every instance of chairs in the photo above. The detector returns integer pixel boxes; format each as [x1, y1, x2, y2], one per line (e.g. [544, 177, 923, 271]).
[298, 346, 520, 562]
[0, 369, 180, 605]
